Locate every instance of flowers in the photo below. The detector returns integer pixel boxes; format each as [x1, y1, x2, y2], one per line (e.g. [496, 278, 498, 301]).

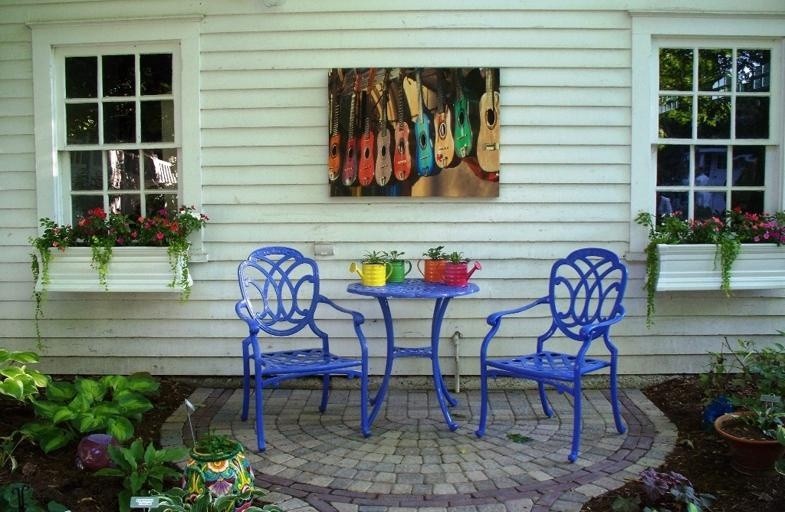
[28, 202, 209, 350]
[631, 198, 784, 330]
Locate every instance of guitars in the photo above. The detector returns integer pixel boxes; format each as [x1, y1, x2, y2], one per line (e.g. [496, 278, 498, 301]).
[329, 69, 501, 189]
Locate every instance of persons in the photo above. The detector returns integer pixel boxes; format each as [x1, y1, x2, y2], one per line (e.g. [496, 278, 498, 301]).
[657, 166, 712, 220]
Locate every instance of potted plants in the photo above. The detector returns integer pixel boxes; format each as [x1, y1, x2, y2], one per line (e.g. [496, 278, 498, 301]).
[349, 245, 482, 287]
[699, 328, 785, 476]
[184, 434, 254, 511]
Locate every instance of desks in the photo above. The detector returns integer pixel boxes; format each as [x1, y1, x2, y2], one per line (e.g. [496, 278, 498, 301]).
[346, 277, 479, 433]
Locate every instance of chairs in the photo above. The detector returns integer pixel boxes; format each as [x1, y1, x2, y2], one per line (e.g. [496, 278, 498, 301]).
[234, 246, 371, 453]
[476, 246, 628, 464]
